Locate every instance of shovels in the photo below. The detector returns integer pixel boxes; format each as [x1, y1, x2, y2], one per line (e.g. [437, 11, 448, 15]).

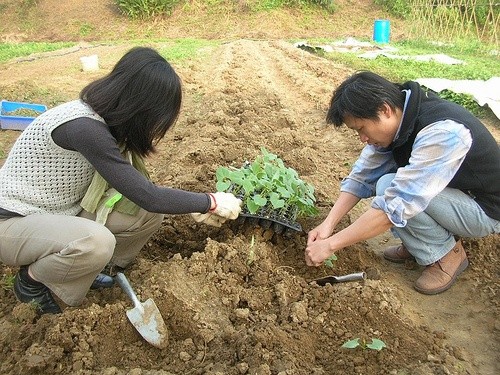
[114, 272, 169, 349]
[313, 271, 367, 285]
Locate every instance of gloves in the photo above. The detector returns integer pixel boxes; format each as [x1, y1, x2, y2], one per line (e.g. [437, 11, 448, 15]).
[209, 191, 243, 220]
[190, 212, 226, 227]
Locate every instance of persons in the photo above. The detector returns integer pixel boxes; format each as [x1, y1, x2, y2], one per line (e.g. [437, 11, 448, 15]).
[305, 71, 500, 295]
[0, 47, 242, 319]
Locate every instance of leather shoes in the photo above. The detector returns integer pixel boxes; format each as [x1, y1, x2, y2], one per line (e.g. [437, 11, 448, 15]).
[90, 273, 114, 290]
[12, 272, 62, 321]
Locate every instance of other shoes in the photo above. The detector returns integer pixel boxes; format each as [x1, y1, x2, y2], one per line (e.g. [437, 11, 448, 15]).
[414, 235, 469, 296]
[384, 242, 414, 263]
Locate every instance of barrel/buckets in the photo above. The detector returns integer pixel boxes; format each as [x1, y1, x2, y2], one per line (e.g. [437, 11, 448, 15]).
[80, 54, 99, 71]
[373, 19, 392, 44]
